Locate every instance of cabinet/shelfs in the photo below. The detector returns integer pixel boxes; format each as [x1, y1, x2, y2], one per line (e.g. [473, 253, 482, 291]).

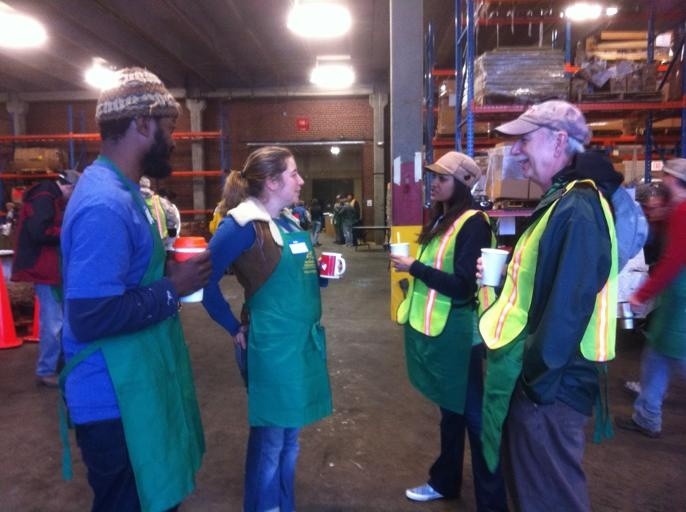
[424, 25, 685, 229]
[1, 99, 229, 247]
[450, 0, 686, 254]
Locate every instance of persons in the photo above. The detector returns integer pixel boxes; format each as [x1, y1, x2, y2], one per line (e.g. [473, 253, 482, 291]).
[387, 150, 509, 512]
[474, 100, 617, 512]
[9, 168, 80, 387]
[606, 159, 685, 438]
[200, 145, 349, 512]
[292, 192, 361, 246]
[210, 198, 234, 275]
[155, 188, 181, 251]
[138, 177, 177, 245]
[59, 66, 213, 512]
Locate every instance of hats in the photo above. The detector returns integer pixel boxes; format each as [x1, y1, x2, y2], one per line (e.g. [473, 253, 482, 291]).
[57, 169, 78, 186]
[95, 66, 177, 120]
[494, 100, 588, 142]
[423, 151, 481, 189]
[662, 158, 685, 182]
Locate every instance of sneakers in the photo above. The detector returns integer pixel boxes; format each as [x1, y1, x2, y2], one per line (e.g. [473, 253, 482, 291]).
[624, 382, 641, 399]
[32, 377, 59, 388]
[614, 414, 661, 438]
[405, 483, 443, 501]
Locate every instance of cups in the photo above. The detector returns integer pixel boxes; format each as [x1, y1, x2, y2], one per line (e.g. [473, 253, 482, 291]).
[481, 248, 510, 287]
[619, 302, 634, 330]
[390, 243, 410, 257]
[173, 236, 209, 302]
[320, 252, 346, 279]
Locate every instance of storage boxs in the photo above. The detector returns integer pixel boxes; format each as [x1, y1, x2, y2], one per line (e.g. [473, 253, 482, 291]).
[435, 79, 491, 136]
[12, 147, 68, 175]
[485, 143, 545, 201]
